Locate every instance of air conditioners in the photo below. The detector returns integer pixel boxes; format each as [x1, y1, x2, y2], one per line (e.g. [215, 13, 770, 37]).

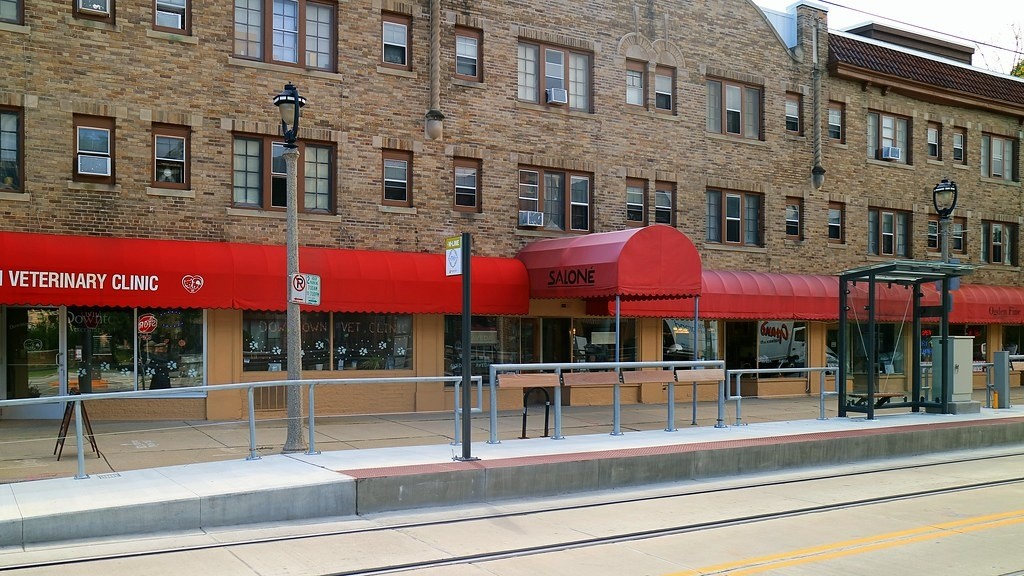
[519, 210, 544, 227]
[547, 88, 568, 105]
[883, 147, 900, 160]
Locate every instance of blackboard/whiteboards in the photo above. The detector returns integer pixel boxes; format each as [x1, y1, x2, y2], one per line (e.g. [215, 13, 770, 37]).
[49, 387, 100, 462]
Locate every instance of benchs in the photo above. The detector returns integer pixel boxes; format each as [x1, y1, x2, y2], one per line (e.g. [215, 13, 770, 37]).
[849, 394, 903, 407]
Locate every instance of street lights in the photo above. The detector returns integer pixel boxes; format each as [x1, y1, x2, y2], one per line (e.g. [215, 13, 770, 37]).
[271, 80, 310, 453]
[932, 177, 958, 415]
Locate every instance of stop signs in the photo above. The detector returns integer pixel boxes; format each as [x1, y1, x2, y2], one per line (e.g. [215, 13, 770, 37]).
[138, 315, 157, 334]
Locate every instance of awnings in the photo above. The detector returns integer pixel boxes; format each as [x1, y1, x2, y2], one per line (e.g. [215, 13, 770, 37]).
[585, 270, 1024, 324]
[0, 230, 531, 316]
[514, 225, 702, 434]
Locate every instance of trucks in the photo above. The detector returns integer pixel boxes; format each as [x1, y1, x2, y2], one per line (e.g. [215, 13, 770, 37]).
[756, 320, 807, 368]
